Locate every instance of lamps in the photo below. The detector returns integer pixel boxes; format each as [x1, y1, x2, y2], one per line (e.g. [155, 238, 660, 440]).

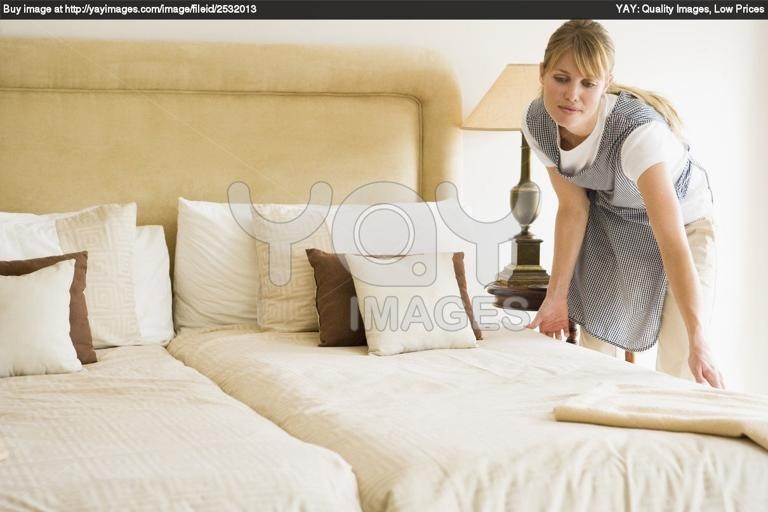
[457, 62, 555, 292]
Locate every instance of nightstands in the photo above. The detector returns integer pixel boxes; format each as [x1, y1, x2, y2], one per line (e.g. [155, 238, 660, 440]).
[484, 285, 578, 347]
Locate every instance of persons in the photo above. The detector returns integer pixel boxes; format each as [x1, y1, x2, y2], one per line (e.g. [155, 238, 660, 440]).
[526, 20, 727, 391]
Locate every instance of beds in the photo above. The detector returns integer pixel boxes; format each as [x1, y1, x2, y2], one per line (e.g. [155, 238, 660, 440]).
[1, 201, 363, 512]
[167, 194, 768, 512]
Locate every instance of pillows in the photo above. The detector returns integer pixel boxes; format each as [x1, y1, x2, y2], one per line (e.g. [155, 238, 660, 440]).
[304, 246, 483, 349]
[345, 249, 478, 356]
[245, 198, 469, 335]
[2, 201, 176, 377]
